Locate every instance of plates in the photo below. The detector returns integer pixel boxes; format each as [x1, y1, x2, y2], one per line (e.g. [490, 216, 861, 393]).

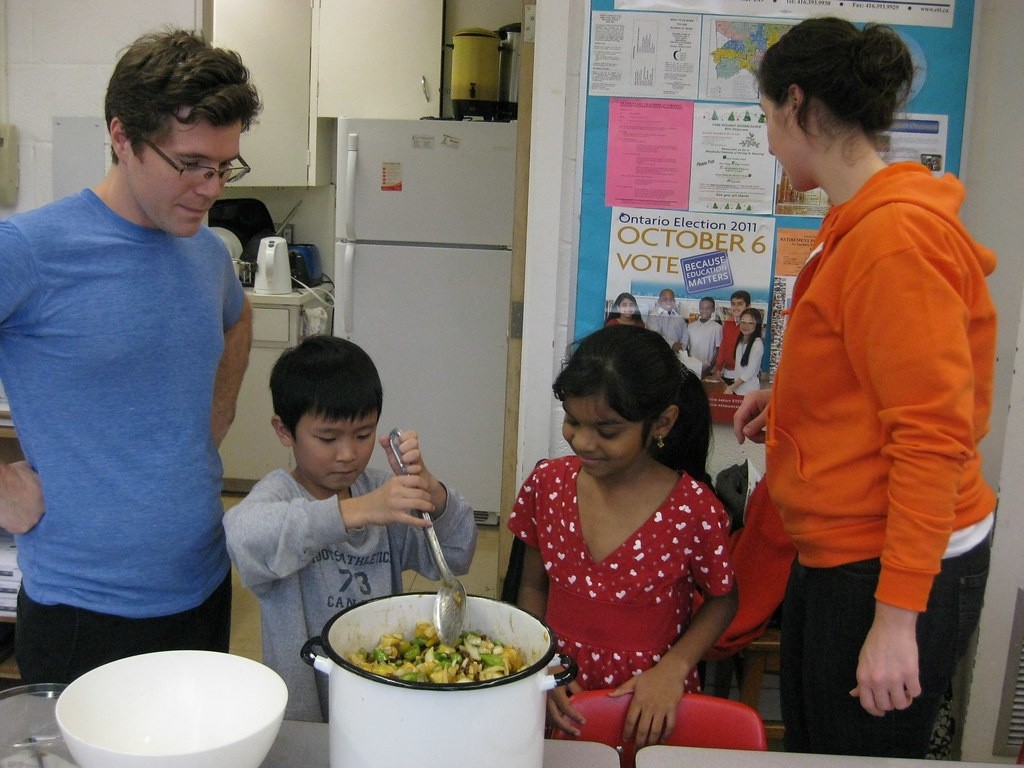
[205, 225, 243, 261]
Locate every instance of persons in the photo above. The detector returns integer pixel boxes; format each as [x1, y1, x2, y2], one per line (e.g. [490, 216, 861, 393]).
[508, 327, 741, 750]
[732, 15, 997, 768]
[219, 333, 476, 723]
[0, 28, 253, 685]
[605, 288, 763, 400]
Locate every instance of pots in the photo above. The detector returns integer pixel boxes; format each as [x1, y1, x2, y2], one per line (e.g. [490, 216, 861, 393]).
[298, 591, 578, 768]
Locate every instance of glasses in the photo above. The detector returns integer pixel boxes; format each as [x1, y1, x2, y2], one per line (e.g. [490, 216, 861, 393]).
[141, 133, 251, 183]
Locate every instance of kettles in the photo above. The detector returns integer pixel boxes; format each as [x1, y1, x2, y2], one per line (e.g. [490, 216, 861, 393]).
[444, 21, 525, 120]
[251, 236, 294, 294]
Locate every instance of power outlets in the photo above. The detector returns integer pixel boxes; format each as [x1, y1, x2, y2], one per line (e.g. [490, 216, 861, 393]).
[284, 229, 292, 243]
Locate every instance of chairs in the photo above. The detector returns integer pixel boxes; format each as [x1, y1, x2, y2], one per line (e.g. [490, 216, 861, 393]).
[550, 688, 766, 768]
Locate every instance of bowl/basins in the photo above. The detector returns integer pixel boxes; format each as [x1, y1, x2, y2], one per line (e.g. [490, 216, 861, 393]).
[0, 683, 79, 768]
[55, 650, 288, 768]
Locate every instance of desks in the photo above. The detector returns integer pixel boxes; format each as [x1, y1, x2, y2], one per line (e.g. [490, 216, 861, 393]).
[221, 281, 330, 493]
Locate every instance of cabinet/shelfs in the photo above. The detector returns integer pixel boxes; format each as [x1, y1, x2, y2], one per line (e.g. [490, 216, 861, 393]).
[195, 0, 317, 186]
[318, 0, 448, 120]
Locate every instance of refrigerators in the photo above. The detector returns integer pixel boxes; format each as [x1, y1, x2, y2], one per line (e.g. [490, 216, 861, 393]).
[331, 115, 517, 530]
